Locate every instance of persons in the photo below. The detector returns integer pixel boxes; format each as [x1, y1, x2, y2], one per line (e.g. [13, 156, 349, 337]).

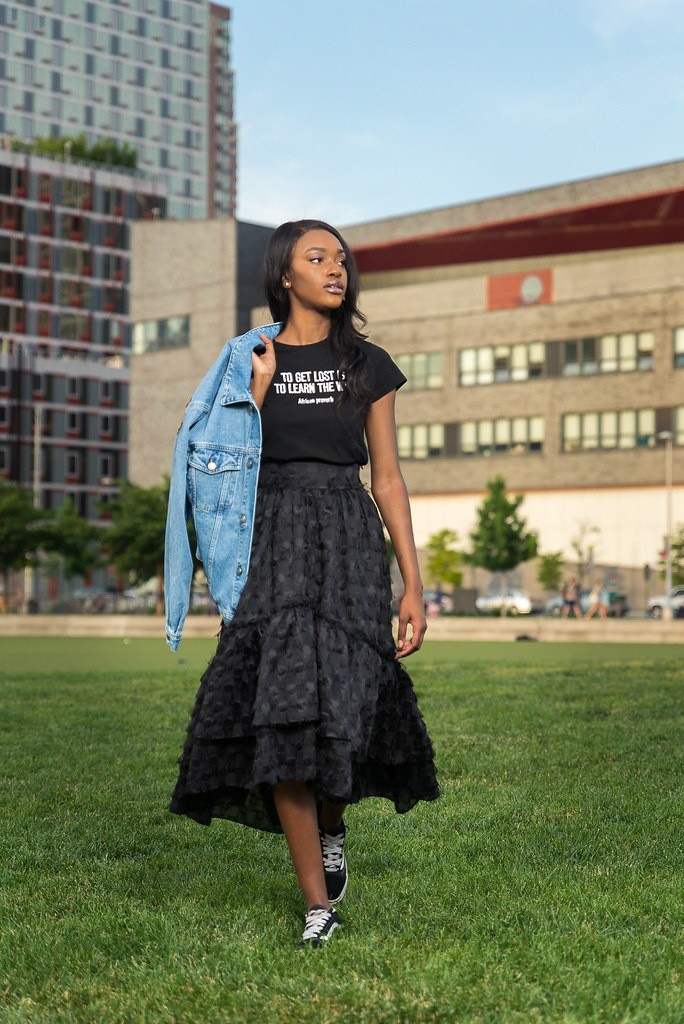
[584, 580, 609, 620]
[165, 218, 444, 948]
[561, 579, 582, 619]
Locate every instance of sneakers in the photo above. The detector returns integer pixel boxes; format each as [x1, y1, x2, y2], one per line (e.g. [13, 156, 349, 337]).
[301, 905, 344, 948]
[317, 816, 348, 904]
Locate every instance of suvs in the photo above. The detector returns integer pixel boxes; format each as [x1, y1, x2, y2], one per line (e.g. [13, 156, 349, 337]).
[646, 584, 684, 620]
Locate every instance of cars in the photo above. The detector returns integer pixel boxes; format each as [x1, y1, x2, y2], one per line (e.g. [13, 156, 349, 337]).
[472, 588, 546, 617]
[389, 590, 454, 617]
[545, 587, 628, 619]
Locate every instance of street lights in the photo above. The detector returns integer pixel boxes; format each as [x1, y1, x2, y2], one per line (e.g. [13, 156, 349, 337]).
[657, 429, 674, 622]
